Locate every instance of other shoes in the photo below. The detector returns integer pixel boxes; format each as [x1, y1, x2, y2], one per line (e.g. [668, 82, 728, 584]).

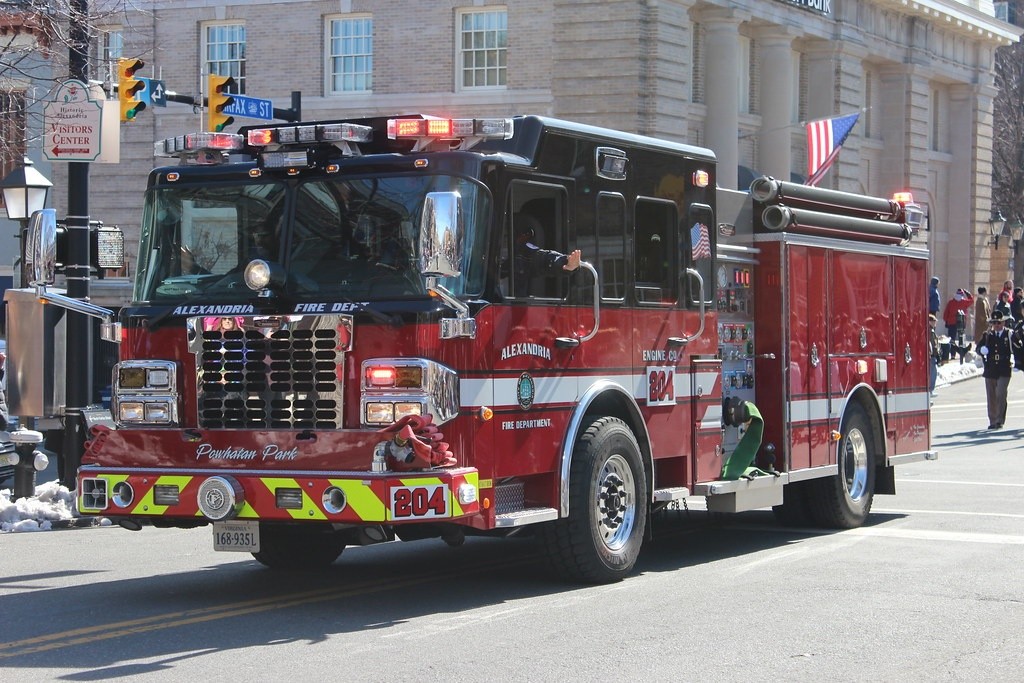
[959, 343, 972, 357]
[951, 356, 955, 360]
[988, 425, 995, 429]
[996, 423, 1003, 429]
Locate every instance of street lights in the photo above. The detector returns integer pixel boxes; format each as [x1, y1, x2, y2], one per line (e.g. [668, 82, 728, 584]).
[3, 155, 54, 287]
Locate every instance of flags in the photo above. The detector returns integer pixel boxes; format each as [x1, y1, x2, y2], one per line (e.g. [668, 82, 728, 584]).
[806, 112, 863, 186]
[691, 223, 711, 261]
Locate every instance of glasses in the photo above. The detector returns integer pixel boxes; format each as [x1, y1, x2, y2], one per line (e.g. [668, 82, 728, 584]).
[1004, 296, 1010, 298]
[1020, 292, 1023, 293]
[993, 322, 1002, 325]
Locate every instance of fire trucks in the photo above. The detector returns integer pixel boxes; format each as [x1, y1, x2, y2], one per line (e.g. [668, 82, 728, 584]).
[71, 112, 941, 588]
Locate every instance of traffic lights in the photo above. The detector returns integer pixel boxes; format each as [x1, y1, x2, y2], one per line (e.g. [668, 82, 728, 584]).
[116, 56, 148, 124]
[89, 221, 125, 273]
[207, 72, 235, 132]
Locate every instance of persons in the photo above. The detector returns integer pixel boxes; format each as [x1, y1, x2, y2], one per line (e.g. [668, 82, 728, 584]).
[0, 352, 9, 429]
[498, 210, 582, 294]
[350, 209, 409, 295]
[927, 276, 1024, 431]
[181, 215, 281, 276]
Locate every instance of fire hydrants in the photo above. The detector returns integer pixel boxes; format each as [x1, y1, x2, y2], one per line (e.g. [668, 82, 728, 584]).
[0, 423, 48, 501]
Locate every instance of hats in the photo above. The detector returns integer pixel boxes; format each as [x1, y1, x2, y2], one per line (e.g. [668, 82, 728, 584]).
[1014, 287, 1022, 294]
[957, 289, 964, 295]
[986, 310, 1010, 322]
[929, 316, 937, 321]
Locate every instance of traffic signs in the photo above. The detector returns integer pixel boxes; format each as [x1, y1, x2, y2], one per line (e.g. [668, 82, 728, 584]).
[40, 80, 104, 163]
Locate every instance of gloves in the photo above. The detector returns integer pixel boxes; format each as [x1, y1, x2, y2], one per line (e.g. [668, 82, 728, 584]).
[945, 324, 949, 328]
[980, 346, 988, 355]
[963, 288, 971, 296]
